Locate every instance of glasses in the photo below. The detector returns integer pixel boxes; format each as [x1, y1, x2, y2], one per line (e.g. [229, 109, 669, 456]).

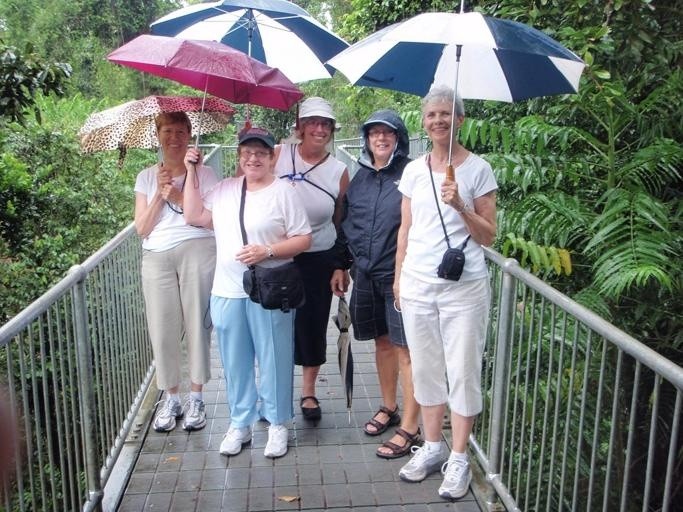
[368, 129, 397, 136]
[239, 151, 272, 158]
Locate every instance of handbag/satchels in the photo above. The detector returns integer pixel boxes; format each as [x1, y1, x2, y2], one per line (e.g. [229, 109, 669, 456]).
[242, 260, 309, 314]
[437, 247, 466, 281]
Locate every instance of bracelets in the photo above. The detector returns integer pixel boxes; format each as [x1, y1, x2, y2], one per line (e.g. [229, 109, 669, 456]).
[459, 203, 467, 215]
[393, 300, 402, 313]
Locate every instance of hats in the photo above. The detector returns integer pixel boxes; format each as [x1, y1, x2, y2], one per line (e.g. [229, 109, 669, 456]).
[237, 127, 276, 150]
[297, 97, 339, 131]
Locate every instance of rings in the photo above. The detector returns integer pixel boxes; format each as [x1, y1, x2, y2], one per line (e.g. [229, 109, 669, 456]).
[442, 192, 444, 196]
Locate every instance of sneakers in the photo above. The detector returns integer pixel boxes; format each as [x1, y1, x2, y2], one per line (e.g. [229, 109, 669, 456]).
[152, 396, 185, 432]
[217, 422, 252, 456]
[263, 423, 289, 459]
[437, 452, 474, 500]
[179, 396, 208, 432]
[398, 442, 448, 483]
[299, 395, 323, 422]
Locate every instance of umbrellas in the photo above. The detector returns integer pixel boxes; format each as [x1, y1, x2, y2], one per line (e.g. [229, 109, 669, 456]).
[322, 0, 588, 180]
[105, 34, 304, 165]
[77, 96, 234, 167]
[149, 0, 351, 128]
[331, 285, 353, 424]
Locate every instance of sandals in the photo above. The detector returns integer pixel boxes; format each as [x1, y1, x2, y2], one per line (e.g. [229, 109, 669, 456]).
[361, 403, 401, 436]
[375, 425, 422, 459]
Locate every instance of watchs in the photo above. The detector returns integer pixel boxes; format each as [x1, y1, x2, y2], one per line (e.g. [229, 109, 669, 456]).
[266, 246, 273, 259]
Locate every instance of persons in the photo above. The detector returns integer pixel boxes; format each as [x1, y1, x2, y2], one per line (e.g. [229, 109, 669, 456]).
[133, 112, 217, 433]
[330, 109, 421, 460]
[392, 84, 499, 498]
[182, 128, 313, 459]
[236, 96, 349, 421]
